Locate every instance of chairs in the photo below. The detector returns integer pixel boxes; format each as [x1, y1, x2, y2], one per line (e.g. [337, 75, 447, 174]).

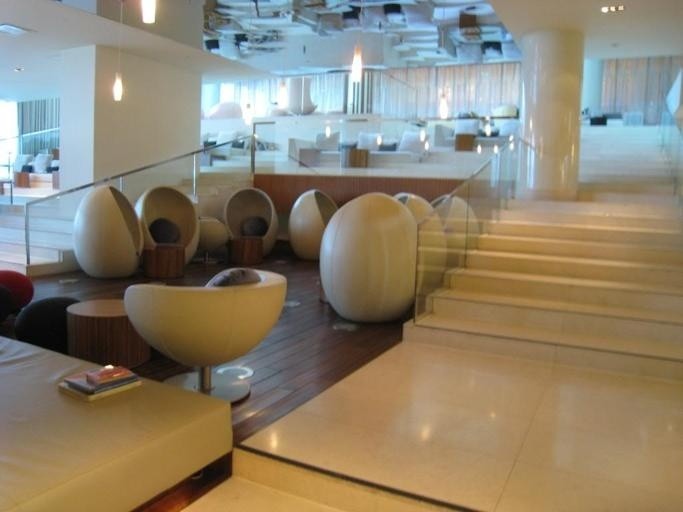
[10, 147, 59, 191]
[123, 265, 288, 408]
[291, 190, 479, 325]
[71, 189, 278, 278]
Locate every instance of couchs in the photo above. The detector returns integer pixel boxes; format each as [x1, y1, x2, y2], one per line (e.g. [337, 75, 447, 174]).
[209, 118, 518, 167]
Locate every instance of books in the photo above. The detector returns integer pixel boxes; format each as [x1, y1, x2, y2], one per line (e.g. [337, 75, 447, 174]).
[58, 366, 142, 402]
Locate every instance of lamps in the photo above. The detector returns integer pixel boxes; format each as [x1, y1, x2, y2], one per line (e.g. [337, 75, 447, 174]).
[439, 0, 448, 120]
[240, 0, 287, 125]
[350, 0, 364, 83]
[113, 0, 123, 101]
[141, 0, 156, 24]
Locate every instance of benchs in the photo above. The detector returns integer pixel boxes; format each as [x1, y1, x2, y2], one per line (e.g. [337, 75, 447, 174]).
[0, 333, 232, 512]
